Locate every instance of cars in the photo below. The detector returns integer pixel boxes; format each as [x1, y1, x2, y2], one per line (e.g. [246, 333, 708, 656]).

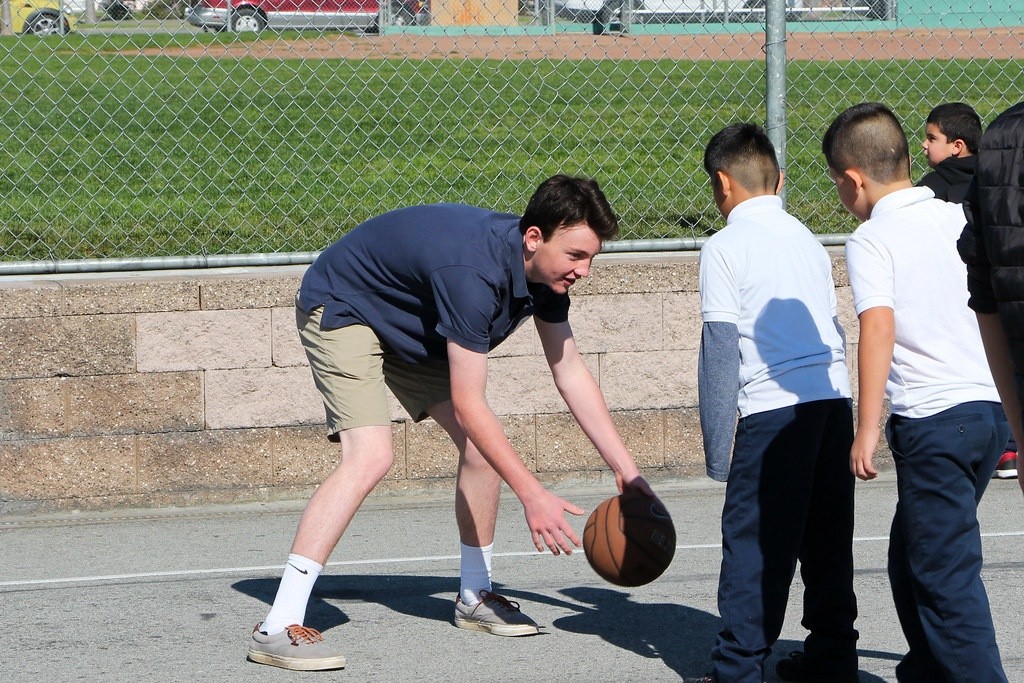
[0, 1, 151, 39]
[183, 0, 430, 33]
[518, 0, 897, 35]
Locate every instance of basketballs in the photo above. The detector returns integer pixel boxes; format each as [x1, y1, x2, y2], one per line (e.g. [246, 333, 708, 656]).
[583, 494, 677, 588]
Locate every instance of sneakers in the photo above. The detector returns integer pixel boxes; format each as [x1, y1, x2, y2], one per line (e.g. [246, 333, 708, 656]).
[685, 672, 711, 683]
[456, 589, 538, 636]
[249, 621, 346, 672]
[776, 649, 858, 683]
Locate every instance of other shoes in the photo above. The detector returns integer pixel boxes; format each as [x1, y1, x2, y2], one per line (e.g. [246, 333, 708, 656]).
[992, 452, 1017, 479]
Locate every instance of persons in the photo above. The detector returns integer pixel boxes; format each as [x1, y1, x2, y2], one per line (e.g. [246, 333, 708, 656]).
[916, 101, 1024, 493]
[823, 102, 1010, 683]
[248, 175, 658, 670]
[684, 121, 859, 683]
